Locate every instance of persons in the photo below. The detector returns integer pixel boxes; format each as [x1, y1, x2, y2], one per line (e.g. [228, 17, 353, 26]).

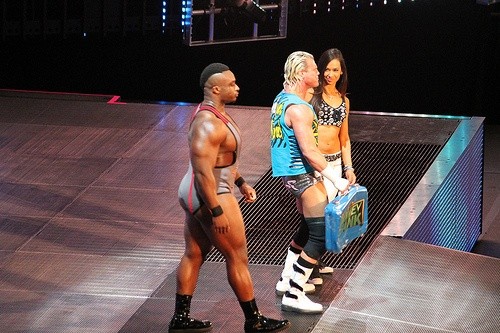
[307, 48, 357, 286]
[269, 50, 352, 316]
[167, 62, 292, 333]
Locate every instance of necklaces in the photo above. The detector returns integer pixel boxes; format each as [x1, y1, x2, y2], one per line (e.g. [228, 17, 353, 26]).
[328, 94, 333, 101]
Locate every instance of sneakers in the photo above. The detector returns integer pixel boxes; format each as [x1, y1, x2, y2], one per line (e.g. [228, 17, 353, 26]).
[308, 265, 334, 285]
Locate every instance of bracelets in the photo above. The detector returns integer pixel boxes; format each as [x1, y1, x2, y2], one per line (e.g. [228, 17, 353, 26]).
[343, 164, 355, 172]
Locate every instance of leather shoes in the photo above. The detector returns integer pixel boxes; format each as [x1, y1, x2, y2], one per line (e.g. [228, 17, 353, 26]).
[167, 310, 290, 333]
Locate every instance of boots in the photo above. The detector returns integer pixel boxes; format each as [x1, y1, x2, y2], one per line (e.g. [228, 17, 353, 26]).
[276, 250, 325, 315]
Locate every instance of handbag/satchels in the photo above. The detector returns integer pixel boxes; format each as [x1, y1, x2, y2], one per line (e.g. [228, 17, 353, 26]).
[324, 183, 368, 254]
